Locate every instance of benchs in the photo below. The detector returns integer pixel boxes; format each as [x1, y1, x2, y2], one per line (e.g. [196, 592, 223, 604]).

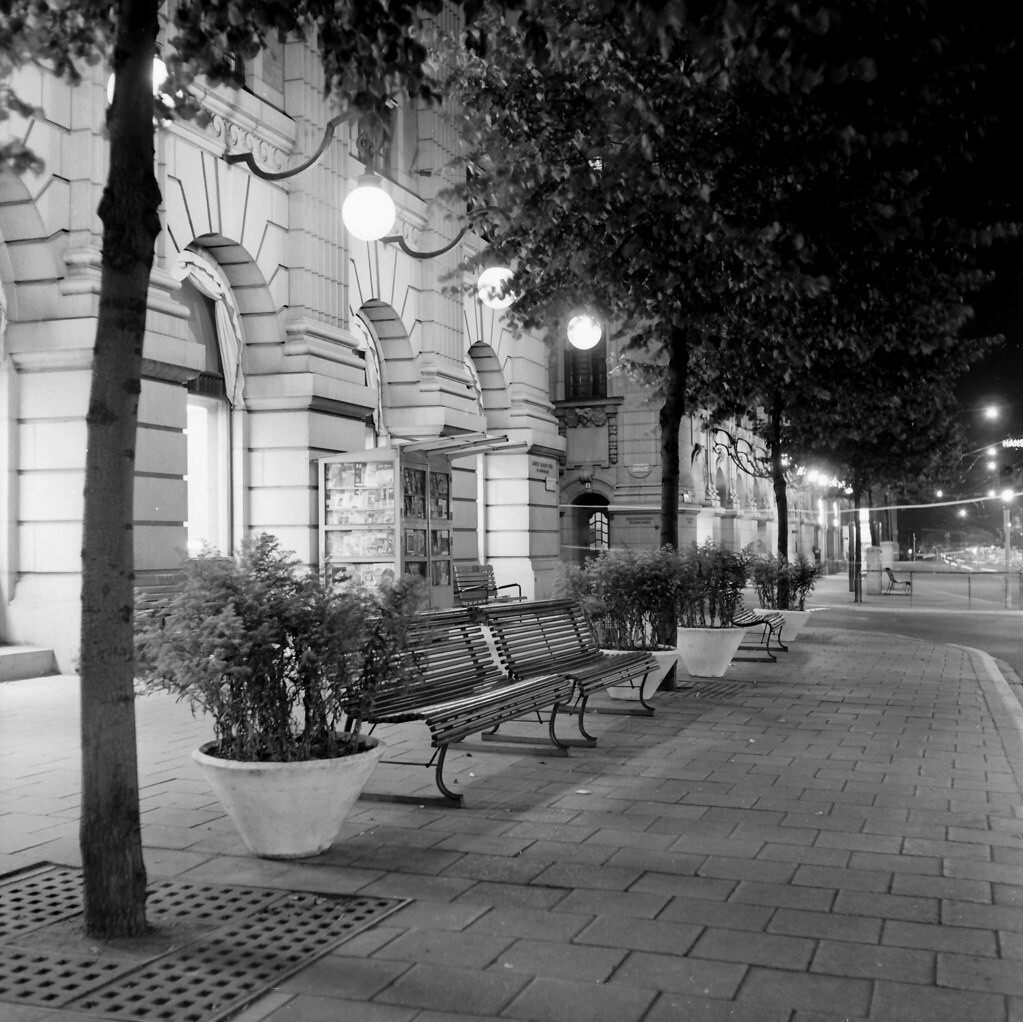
[883, 568, 911, 595]
[467, 598, 660, 748]
[327, 608, 573, 811]
[723, 588, 788, 663]
[454, 565, 528, 606]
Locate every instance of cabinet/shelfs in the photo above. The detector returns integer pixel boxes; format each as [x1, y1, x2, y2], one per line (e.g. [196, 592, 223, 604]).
[324, 463, 452, 610]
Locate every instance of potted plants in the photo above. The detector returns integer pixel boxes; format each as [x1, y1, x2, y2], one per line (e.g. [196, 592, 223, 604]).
[134, 531, 441, 860]
[552, 540, 694, 702]
[748, 551, 827, 641]
[677, 537, 757, 678]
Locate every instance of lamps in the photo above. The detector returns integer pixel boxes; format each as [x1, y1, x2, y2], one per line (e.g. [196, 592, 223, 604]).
[379, 208, 517, 310]
[220, 88, 399, 243]
